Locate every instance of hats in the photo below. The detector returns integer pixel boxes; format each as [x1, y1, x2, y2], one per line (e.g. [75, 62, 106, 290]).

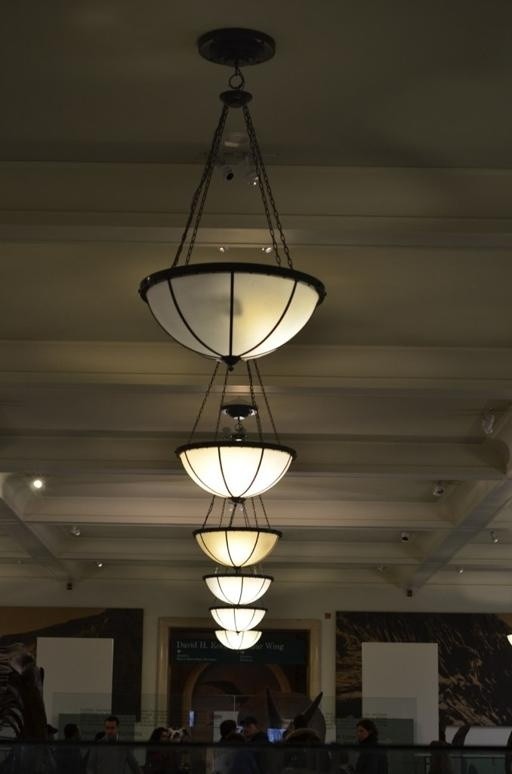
[239, 715, 257, 726]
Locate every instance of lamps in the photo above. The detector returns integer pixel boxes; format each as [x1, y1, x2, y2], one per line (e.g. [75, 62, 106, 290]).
[193, 494, 283, 571]
[137, 26, 327, 370]
[207, 599, 268, 652]
[202, 562, 274, 607]
[173, 360, 297, 507]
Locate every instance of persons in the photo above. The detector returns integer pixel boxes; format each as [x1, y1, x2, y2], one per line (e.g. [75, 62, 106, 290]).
[429, 740, 453, 774]
[47, 713, 388, 774]
